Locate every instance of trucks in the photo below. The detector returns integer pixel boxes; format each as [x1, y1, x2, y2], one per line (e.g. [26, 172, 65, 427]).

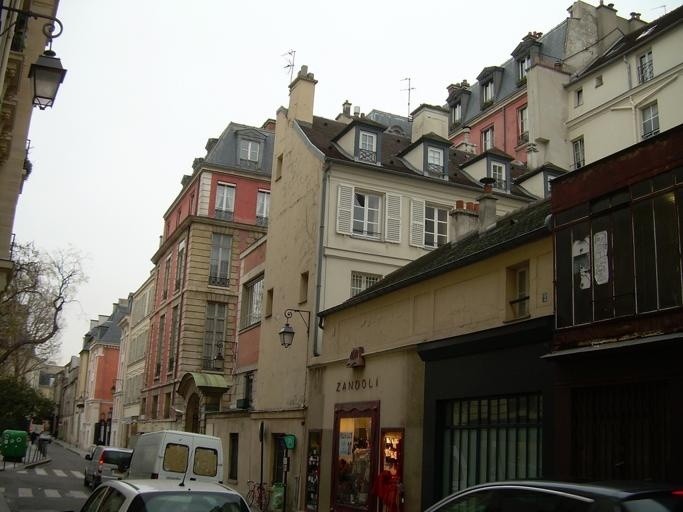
[128, 430, 224, 486]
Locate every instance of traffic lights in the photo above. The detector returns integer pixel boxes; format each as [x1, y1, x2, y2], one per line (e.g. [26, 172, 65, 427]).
[273, 436, 294, 450]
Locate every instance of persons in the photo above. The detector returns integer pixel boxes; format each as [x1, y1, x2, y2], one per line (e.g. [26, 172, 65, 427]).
[30, 430, 52, 453]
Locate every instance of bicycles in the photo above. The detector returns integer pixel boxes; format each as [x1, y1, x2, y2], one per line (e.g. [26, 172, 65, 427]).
[246, 481, 267, 511]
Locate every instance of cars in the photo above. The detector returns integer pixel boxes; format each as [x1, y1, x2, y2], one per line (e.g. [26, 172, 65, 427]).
[424, 480, 683, 512]
[37, 431, 52, 444]
[79, 451, 252, 512]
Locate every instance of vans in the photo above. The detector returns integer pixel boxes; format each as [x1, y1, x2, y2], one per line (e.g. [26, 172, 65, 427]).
[83, 446, 132, 490]
[26, 417, 45, 436]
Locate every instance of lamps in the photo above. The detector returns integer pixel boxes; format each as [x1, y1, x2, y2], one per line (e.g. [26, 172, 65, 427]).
[1, 6, 67, 110]
[278, 308, 311, 348]
[110, 378, 121, 393]
[212, 341, 237, 370]
